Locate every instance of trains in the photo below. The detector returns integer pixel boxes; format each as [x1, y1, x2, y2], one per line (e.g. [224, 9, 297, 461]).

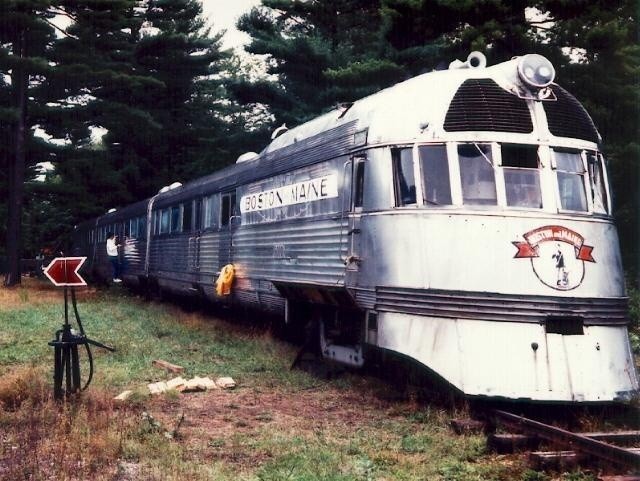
[59, 50, 639, 409]
